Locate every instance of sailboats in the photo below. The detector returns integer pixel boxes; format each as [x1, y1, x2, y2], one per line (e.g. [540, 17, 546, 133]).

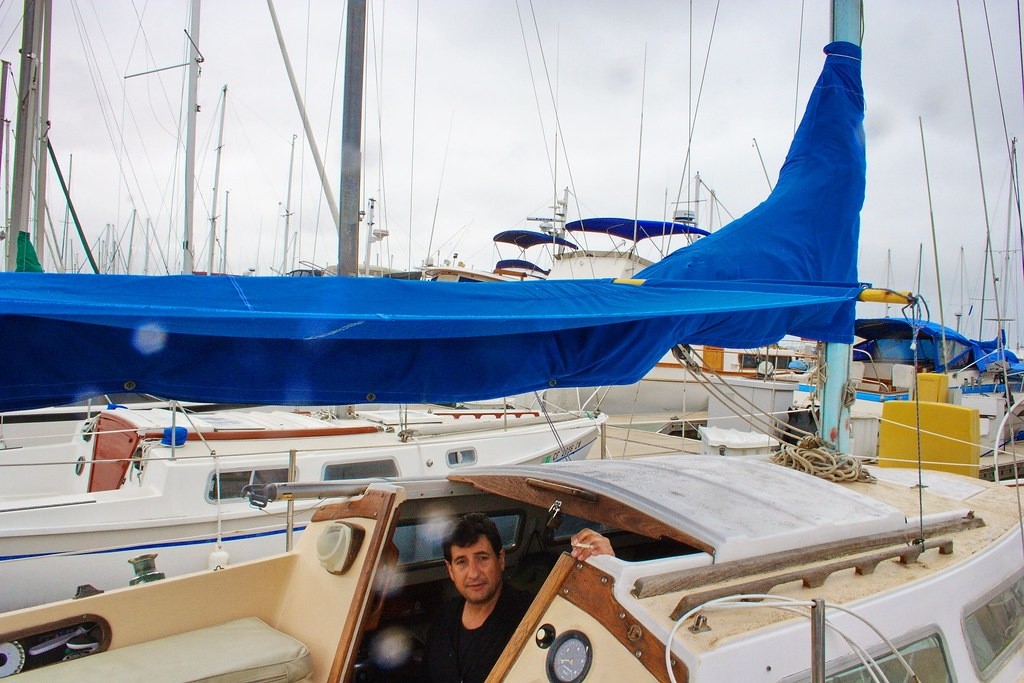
[0, 0, 1022, 683]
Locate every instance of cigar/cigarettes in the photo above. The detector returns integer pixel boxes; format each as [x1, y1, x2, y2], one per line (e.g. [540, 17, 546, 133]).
[571, 542, 594, 549]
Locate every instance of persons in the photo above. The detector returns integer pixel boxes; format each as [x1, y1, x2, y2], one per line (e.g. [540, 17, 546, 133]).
[415, 514, 614, 683]
[1003, 387, 1014, 415]
